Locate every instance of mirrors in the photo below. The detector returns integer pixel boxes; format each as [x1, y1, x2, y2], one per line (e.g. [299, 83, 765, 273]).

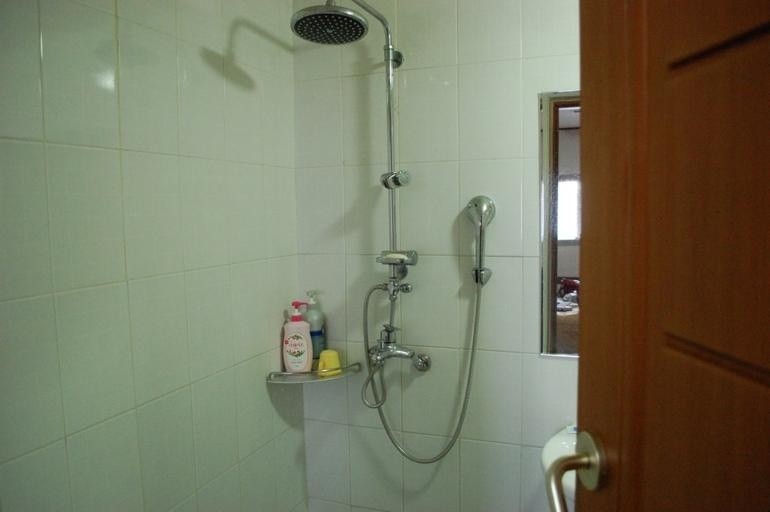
[538, 90, 579, 357]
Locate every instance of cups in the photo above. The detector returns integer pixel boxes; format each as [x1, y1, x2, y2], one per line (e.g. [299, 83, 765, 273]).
[318, 349, 342, 377]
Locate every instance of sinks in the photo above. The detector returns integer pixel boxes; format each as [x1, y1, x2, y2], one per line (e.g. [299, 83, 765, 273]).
[539, 424, 577, 511]
